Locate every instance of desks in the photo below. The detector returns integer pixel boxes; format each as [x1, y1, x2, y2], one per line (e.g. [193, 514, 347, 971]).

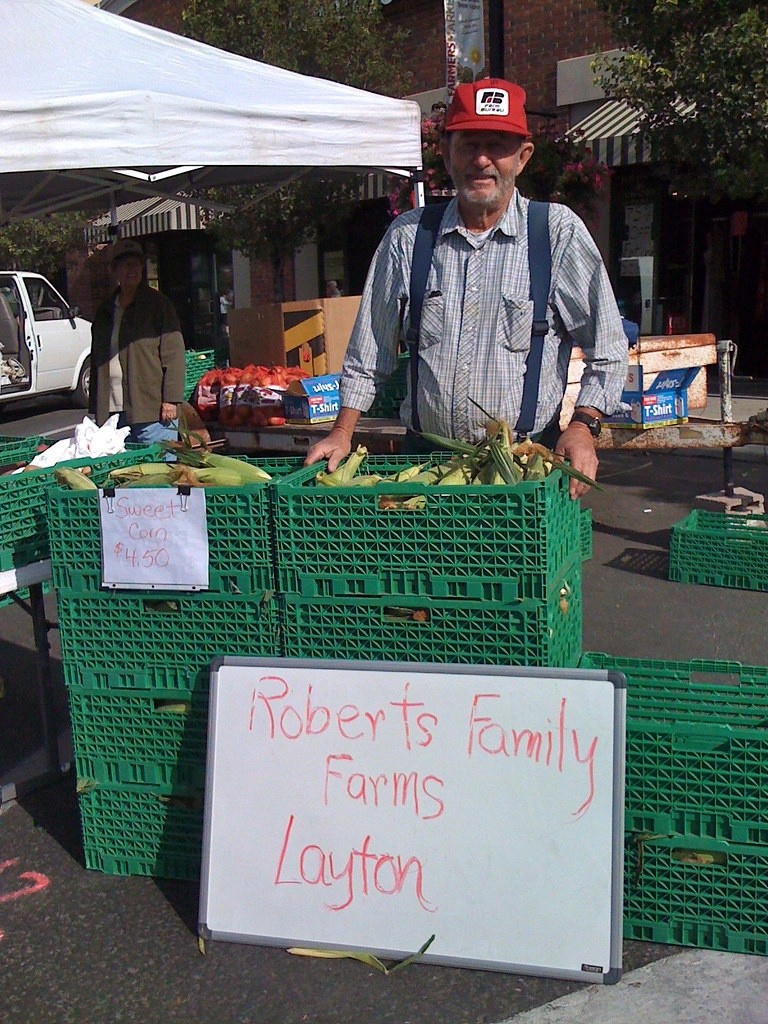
[0, 560, 72, 806]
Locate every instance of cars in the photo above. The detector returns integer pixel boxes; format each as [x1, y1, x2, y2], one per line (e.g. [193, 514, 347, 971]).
[0, 270, 92, 409]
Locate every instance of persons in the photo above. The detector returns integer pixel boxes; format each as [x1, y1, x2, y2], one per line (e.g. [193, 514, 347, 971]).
[220, 291, 234, 333]
[304, 80, 629, 500]
[327, 280, 340, 297]
[88, 240, 185, 462]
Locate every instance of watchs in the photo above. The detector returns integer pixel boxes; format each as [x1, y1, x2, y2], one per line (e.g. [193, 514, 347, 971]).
[568, 411, 602, 438]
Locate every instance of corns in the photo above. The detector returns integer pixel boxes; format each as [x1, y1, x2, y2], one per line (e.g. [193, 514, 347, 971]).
[55, 454, 570, 712]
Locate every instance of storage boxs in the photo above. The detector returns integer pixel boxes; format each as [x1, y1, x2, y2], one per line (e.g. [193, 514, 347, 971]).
[273, 372, 344, 425]
[184, 348, 214, 401]
[603, 366, 700, 429]
[0, 429, 768, 961]
[362, 350, 409, 418]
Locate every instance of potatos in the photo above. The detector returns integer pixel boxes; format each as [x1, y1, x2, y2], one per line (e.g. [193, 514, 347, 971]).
[198, 368, 307, 425]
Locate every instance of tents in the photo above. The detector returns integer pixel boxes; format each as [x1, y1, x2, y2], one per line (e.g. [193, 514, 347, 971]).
[0, 0, 425, 207]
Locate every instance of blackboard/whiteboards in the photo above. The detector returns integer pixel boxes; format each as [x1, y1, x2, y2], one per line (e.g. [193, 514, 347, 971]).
[199, 654, 627, 985]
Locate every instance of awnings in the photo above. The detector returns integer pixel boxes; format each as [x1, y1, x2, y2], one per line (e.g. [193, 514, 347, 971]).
[84, 173, 458, 244]
[555, 87, 768, 168]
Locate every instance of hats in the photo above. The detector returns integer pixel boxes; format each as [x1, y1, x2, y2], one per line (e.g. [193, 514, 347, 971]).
[445, 78, 533, 137]
[112, 239, 143, 257]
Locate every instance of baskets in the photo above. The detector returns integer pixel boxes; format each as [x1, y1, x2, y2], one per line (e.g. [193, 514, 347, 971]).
[184, 349, 216, 400]
[669, 508, 767, 591]
[48, 450, 768, 954]
[0, 434, 167, 570]
